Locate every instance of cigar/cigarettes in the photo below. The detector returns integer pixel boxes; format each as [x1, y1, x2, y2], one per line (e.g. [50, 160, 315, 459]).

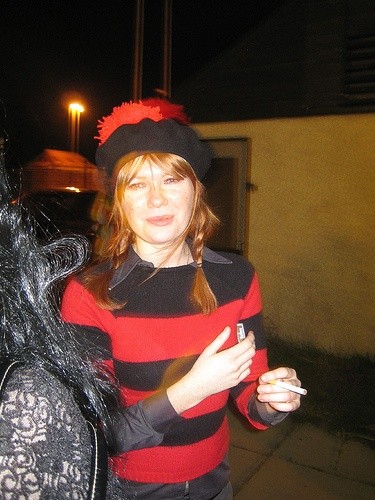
[267, 380, 307, 396]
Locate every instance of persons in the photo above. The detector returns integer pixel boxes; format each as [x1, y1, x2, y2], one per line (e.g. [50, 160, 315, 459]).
[0, 164, 130, 500]
[60, 104, 301, 500]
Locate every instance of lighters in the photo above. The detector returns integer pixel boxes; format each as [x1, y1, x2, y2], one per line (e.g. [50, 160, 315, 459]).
[236, 322, 246, 344]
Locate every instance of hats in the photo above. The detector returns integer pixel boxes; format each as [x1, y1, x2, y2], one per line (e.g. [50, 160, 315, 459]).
[94, 98, 220, 186]
